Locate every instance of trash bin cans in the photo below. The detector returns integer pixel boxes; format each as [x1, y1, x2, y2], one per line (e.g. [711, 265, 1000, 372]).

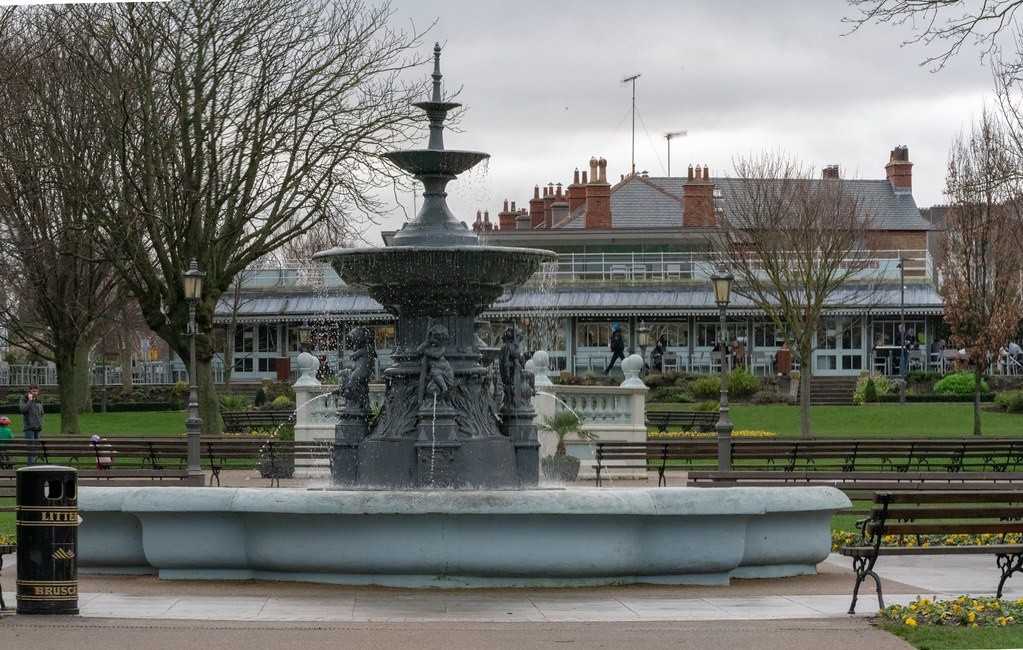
[15, 465, 81, 615]
[275, 357, 291, 381]
[777, 349, 792, 373]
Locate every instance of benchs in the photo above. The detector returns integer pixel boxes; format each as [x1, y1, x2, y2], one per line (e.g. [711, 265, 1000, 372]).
[0, 470, 188, 513]
[687, 470, 1023, 547]
[646, 410, 721, 433]
[591, 437, 1023, 487]
[839, 489, 1023, 614]
[0, 434, 334, 489]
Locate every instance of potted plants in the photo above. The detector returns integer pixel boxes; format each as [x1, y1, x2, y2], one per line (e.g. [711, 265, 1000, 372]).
[535, 408, 599, 482]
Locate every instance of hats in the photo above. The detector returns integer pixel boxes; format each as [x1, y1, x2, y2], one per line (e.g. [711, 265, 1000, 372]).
[0, 416, 11, 425]
[613, 323, 620, 330]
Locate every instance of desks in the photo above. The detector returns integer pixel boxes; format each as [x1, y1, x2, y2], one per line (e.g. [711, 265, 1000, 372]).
[662, 355, 682, 375]
[588, 355, 607, 374]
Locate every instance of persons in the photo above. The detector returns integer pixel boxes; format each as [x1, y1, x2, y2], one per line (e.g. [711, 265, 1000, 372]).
[600, 323, 625, 376]
[20, 384, 44, 467]
[0, 416, 15, 471]
[712, 340, 745, 371]
[872, 324, 947, 375]
[89, 435, 117, 471]
[416, 325, 455, 396]
[651, 340, 667, 371]
[500, 328, 532, 398]
[317, 355, 330, 377]
[347, 326, 375, 387]
[997, 341, 1023, 375]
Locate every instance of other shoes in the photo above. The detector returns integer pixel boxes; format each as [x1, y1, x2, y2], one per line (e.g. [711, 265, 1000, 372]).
[600, 371, 607, 376]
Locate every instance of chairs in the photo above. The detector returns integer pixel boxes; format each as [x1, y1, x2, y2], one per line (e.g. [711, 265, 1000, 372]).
[711, 351, 722, 371]
[909, 349, 923, 371]
[221, 409, 297, 435]
[751, 351, 766, 376]
